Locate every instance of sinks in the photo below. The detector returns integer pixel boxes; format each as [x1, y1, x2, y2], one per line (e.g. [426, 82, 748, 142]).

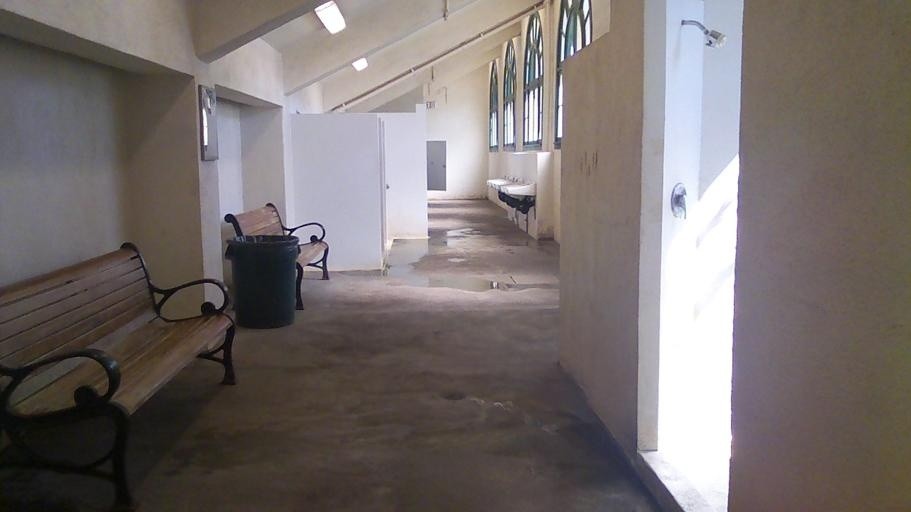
[500, 177, 537, 200]
[487, 178, 514, 189]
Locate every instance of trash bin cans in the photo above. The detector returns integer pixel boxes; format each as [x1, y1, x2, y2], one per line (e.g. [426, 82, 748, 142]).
[225, 234, 300, 328]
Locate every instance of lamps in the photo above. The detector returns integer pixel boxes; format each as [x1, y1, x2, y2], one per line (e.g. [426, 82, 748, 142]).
[315, 1, 346, 36]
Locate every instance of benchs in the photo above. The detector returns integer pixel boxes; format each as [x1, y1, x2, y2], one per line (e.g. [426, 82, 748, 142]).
[0, 241, 237, 510]
[225, 203, 330, 309]
